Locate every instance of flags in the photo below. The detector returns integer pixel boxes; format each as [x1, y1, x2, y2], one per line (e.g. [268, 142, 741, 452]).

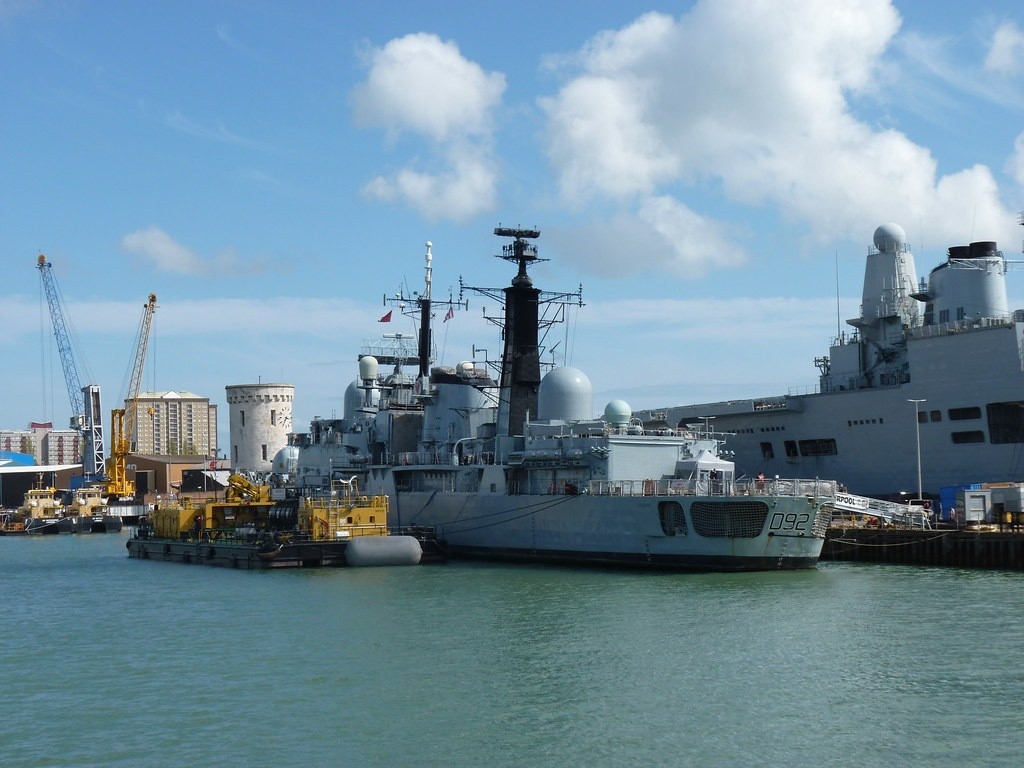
[378, 310, 392, 322]
[443, 308, 454, 323]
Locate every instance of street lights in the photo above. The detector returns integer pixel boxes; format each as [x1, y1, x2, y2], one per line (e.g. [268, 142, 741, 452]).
[698, 416, 716, 440]
[211, 448, 222, 502]
[907, 399, 927, 500]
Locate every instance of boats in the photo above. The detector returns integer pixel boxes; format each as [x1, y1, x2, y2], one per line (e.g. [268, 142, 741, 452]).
[0, 471, 124, 536]
[126, 478, 422, 570]
[268, 222, 935, 571]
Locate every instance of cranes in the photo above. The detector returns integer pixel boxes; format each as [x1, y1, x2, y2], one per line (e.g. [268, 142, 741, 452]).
[35, 256, 159, 501]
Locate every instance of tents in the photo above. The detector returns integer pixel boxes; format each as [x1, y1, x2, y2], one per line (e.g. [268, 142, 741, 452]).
[675, 448, 735, 498]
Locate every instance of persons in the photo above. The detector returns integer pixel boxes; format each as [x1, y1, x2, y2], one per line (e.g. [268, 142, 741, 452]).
[755, 472, 765, 497]
